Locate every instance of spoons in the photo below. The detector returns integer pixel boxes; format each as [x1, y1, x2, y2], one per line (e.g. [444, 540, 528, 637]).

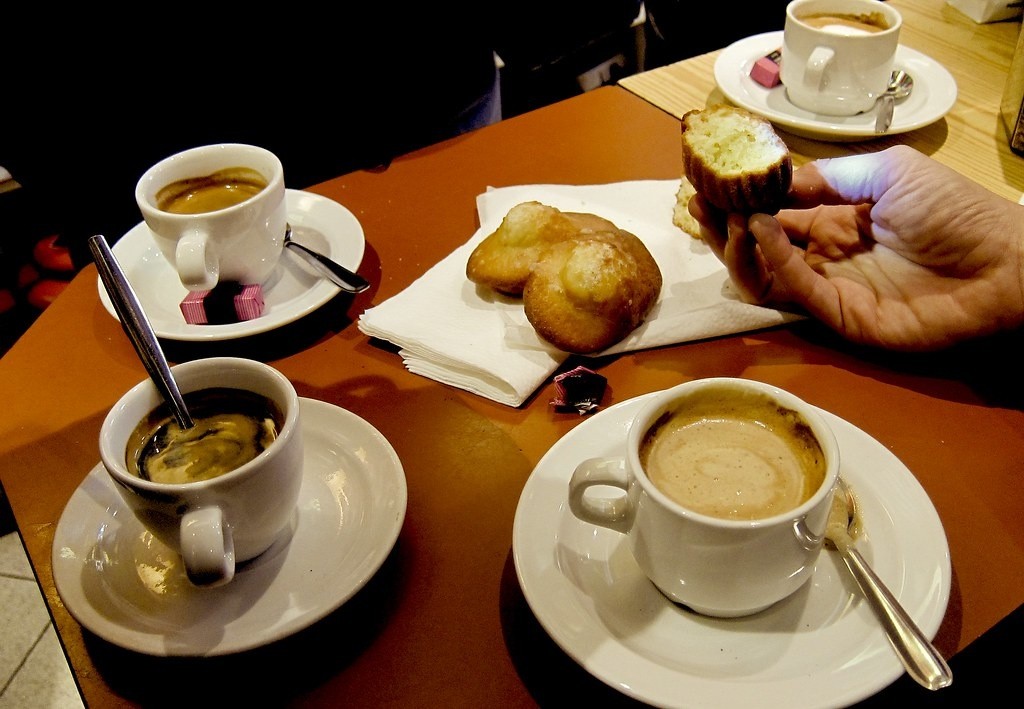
[280, 222, 369, 294]
[875, 70, 913, 128]
[819, 475, 952, 689]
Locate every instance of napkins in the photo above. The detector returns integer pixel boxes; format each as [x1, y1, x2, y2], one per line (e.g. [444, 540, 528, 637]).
[355, 178, 808, 407]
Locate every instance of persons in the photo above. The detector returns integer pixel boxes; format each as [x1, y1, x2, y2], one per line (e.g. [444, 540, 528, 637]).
[686, 143, 1024, 353]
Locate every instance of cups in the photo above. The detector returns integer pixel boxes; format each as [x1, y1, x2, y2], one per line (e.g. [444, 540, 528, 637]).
[568, 378, 841, 621]
[779, 1, 903, 116]
[131, 142, 285, 291]
[99, 356, 313, 589]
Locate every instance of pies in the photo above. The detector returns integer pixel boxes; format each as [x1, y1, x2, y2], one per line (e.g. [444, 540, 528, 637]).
[466, 202, 662, 354]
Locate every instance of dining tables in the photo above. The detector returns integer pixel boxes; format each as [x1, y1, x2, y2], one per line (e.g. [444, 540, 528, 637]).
[1, 1, 1024, 708]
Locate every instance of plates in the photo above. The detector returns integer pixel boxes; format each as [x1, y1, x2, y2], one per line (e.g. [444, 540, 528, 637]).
[512, 388, 951, 709]
[714, 27, 960, 140]
[49, 395, 408, 655]
[97, 188, 365, 341]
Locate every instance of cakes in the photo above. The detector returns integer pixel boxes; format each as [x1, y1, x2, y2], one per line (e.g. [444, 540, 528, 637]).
[681, 104, 791, 215]
